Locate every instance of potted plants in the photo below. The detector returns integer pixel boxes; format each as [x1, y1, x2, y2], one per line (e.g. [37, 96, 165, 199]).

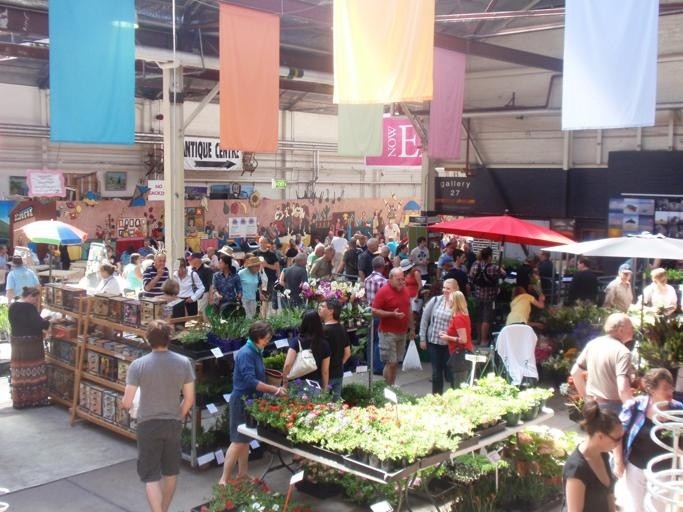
[534, 267, 683, 333]
[638, 312, 683, 388]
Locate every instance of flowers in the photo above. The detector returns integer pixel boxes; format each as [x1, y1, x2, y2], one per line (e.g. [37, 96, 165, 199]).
[198, 334, 585, 512]
[144, 207, 164, 230]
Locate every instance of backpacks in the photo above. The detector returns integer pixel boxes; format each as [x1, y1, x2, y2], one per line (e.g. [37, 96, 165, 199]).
[471, 263, 496, 288]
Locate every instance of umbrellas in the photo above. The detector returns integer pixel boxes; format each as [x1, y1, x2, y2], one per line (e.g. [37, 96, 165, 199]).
[536, 228, 682, 330]
[12, 217, 89, 283]
[424, 211, 578, 321]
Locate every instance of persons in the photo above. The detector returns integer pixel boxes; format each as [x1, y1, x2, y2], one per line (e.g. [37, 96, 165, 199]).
[7, 285, 59, 408]
[564, 256, 599, 305]
[0, 244, 11, 271]
[567, 308, 639, 432]
[636, 268, 678, 316]
[608, 366, 682, 512]
[83, 218, 557, 395]
[217, 319, 289, 487]
[4, 255, 42, 315]
[603, 261, 637, 313]
[117, 320, 194, 511]
[561, 396, 628, 512]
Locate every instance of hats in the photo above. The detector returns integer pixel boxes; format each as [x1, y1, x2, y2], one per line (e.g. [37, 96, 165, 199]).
[217, 245, 235, 258]
[244, 257, 263, 268]
[398, 259, 415, 272]
[619, 263, 633, 274]
[186, 253, 203, 260]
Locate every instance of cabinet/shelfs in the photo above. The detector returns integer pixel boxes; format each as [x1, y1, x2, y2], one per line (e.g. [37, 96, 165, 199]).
[36, 282, 374, 466]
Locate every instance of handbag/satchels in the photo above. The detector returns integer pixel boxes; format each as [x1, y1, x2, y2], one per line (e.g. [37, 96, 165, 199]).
[286, 339, 318, 381]
[191, 270, 203, 300]
[446, 336, 479, 373]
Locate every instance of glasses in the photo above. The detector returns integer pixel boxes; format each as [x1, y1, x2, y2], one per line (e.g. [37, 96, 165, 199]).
[606, 434, 621, 442]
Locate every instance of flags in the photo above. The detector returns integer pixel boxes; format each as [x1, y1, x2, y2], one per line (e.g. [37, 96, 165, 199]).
[216, 4, 284, 157]
[46, 2, 142, 148]
[426, 46, 468, 166]
[330, 0, 436, 109]
[335, 102, 389, 162]
[558, 0, 661, 135]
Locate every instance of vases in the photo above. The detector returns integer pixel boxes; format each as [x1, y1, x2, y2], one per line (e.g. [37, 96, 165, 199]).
[152, 228, 160, 237]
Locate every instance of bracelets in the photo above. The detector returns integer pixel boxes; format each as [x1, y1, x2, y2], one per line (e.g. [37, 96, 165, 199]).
[274, 387, 281, 395]
